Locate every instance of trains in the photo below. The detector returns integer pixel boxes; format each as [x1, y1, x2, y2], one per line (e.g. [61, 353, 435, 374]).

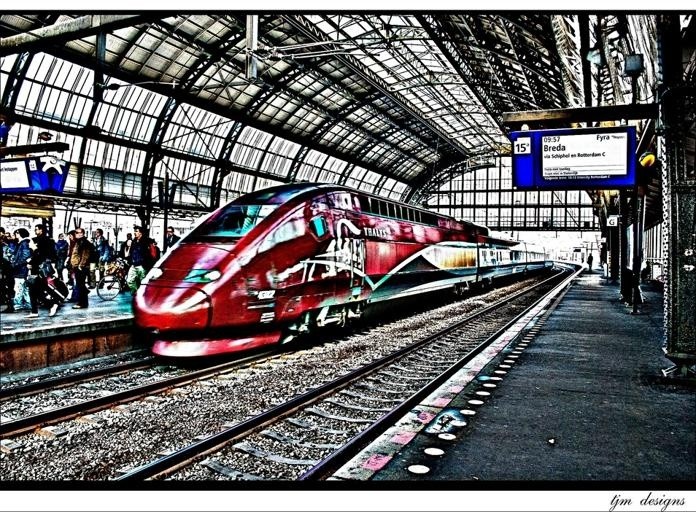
[132, 181, 555, 362]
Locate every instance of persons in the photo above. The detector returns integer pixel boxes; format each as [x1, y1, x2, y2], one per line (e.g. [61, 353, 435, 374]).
[641, 261, 652, 283]
[587, 252, 593, 271]
[0, 222, 182, 321]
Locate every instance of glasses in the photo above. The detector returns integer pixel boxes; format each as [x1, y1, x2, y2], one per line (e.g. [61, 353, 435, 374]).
[75, 231, 83, 234]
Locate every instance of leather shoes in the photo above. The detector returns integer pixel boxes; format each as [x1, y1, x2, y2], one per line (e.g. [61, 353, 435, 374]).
[49, 303, 59, 316]
[72, 304, 88, 309]
[26, 312, 39, 318]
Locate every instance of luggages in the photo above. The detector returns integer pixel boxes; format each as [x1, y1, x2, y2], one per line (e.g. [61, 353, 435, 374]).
[46, 275, 68, 301]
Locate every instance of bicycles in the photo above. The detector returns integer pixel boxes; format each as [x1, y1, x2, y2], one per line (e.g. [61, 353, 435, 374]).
[96, 259, 131, 301]
